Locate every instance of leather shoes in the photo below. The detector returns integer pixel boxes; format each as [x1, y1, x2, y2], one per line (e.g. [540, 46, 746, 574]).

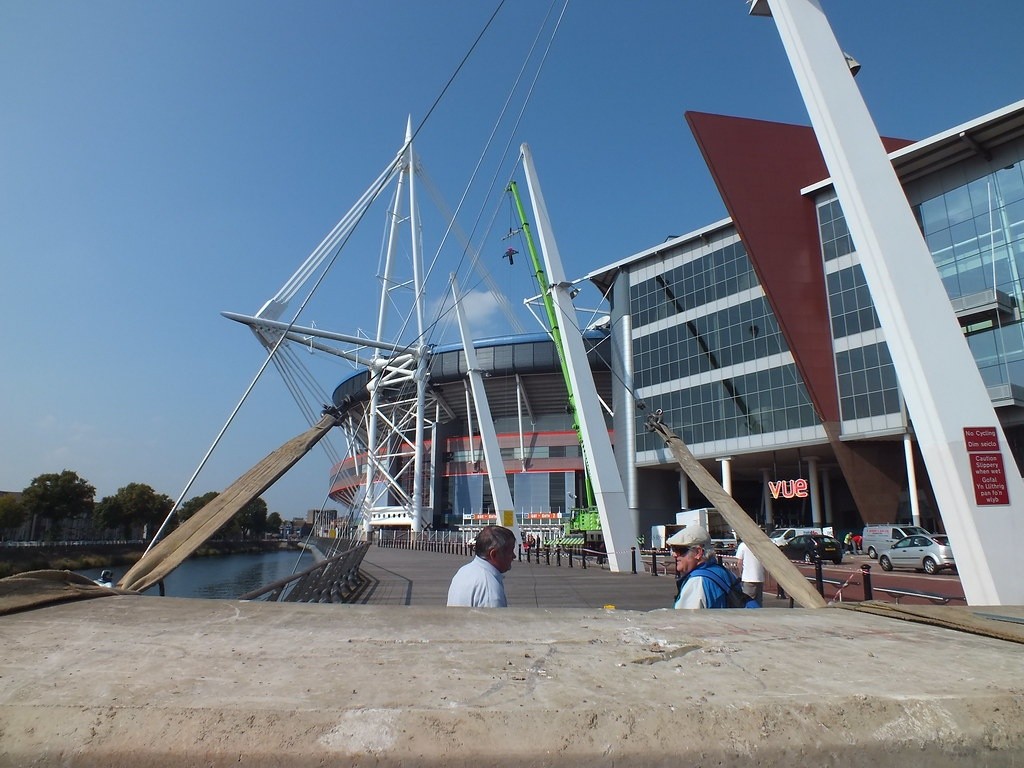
[777, 594, 789, 599]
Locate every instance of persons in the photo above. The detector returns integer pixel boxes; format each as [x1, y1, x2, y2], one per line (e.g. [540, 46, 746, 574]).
[777, 583, 789, 599]
[598, 542, 607, 564]
[666, 525, 742, 609]
[447, 525, 517, 607]
[736, 542, 765, 607]
[843, 532, 853, 554]
[851, 535, 862, 555]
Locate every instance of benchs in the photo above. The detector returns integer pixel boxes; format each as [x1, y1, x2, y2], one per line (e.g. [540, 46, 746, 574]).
[574, 555, 594, 567]
[873, 584, 953, 604]
[640, 559, 674, 575]
[805, 576, 859, 601]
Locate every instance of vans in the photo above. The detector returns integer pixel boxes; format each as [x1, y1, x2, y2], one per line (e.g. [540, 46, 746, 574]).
[862, 522, 938, 559]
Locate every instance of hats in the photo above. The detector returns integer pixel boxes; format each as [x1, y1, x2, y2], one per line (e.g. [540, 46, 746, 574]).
[666, 525, 711, 546]
[849, 532, 853, 535]
[101, 570, 113, 580]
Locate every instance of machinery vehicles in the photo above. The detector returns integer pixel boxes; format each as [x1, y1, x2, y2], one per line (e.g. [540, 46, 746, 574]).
[503, 180, 607, 556]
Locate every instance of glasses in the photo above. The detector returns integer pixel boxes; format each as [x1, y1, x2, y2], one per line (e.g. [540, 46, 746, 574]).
[672, 547, 696, 555]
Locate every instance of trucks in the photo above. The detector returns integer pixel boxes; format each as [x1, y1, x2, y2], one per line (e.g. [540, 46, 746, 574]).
[676, 508, 738, 555]
[651, 525, 687, 552]
[768, 527, 835, 547]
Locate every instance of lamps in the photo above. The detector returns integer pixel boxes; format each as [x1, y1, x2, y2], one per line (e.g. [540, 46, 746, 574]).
[571, 285, 584, 299]
[558, 280, 573, 289]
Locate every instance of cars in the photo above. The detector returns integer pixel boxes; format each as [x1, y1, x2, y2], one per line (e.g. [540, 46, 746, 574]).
[467, 537, 477, 551]
[878, 534, 957, 574]
[778, 535, 842, 565]
